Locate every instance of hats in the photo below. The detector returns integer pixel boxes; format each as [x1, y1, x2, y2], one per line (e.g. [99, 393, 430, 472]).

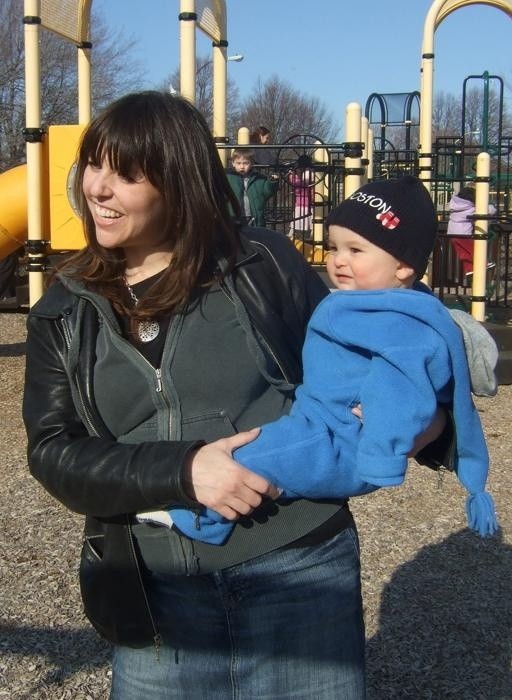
[325, 175, 439, 282]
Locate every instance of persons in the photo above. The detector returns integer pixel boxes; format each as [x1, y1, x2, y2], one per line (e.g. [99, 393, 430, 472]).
[135, 173, 499, 546]
[22, 92, 448, 700]
[447, 185, 496, 276]
[287, 155, 314, 242]
[224, 126, 286, 227]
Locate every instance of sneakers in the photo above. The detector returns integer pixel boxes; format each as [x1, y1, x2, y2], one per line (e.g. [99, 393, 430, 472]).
[464, 261, 496, 276]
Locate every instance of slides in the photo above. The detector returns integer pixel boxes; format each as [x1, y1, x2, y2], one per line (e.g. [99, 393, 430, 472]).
[0, 163, 27, 260]
